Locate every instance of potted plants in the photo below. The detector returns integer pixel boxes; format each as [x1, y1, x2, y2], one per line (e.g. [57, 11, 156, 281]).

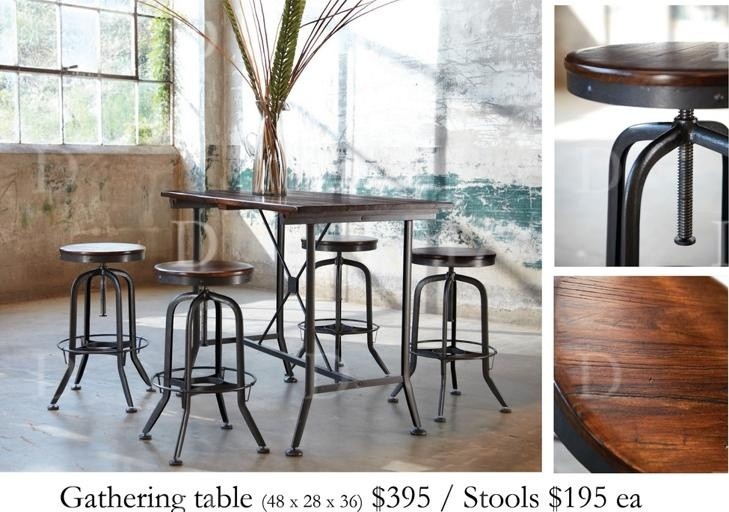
[141, 0, 398, 197]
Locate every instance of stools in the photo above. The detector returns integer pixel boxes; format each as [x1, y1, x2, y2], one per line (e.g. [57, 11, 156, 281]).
[389, 246, 513, 422]
[564, 40, 728, 266]
[289, 235, 391, 377]
[47, 242, 156, 412]
[140, 259, 271, 465]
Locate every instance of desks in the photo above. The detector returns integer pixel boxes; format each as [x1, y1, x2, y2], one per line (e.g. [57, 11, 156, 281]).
[160, 190, 454, 457]
